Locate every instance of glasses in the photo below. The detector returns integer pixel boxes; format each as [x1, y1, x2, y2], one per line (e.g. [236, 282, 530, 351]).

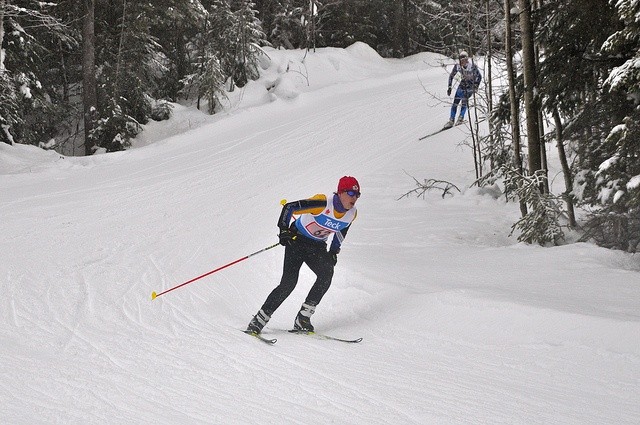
[343, 190, 361, 198]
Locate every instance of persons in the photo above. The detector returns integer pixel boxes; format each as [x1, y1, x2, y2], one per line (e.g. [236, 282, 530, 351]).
[246, 176, 361, 334]
[444, 50, 482, 128]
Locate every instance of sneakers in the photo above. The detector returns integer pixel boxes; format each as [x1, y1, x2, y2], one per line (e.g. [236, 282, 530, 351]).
[455, 116, 464, 126]
[444, 118, 455, 129]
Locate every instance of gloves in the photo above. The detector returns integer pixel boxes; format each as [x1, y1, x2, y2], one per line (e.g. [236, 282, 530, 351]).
[279, 232, 296, 247]
[326, 250, 337, 266]
[447, 88, 452, 96]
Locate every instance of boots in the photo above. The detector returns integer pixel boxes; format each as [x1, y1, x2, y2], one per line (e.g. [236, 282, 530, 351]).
[293, 299, 317, 332]
[248, 309, 271, 335]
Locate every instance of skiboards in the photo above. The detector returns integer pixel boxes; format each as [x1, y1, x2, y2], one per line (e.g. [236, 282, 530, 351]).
[241, 330, 363, 344]
[419, 120, 468, 140]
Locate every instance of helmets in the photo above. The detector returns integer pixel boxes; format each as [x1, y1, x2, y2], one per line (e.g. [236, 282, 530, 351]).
[460, 51, 468, 61]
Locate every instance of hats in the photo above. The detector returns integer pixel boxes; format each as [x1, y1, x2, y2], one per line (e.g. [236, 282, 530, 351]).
[338, 176, 361, 194]
